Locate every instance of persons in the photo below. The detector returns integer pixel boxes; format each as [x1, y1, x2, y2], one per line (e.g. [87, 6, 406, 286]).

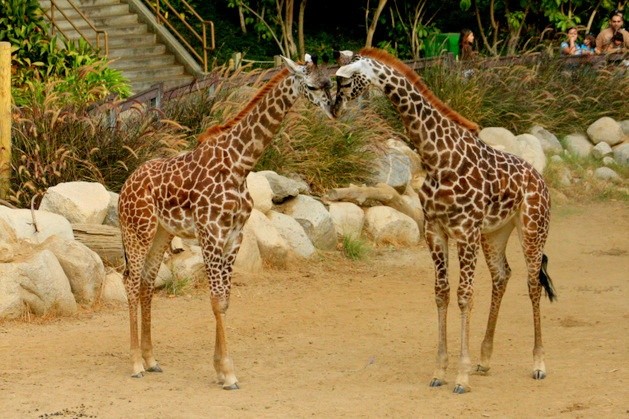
[443, 11, 625, 60]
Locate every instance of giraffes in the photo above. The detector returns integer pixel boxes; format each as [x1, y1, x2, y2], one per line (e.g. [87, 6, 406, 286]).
[331, 48, 560, 395]
[117, 52, 330, 390]
